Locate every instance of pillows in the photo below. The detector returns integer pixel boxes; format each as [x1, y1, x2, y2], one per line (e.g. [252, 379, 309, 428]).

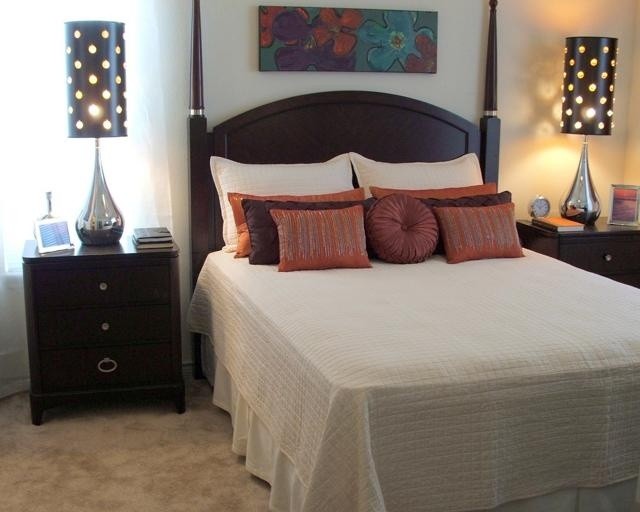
[228, 186, 366, 261]
[348, 150, 484, 199]
[239, 195, 377, 267]
[368, 182, 499, 200]
[207, 152, 357, 254]
[416, 189, 517, 257]
[432, 201, 525, 266]
[364, 192, 441, 265]
[269, 204, 375, 273]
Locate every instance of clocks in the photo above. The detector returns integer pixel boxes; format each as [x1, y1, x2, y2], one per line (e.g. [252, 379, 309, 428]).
[530, 198, 550, 219]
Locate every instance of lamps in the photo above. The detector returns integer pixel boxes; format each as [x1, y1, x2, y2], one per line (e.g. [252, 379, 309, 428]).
[62, 19, 130, 245]
[559, 36, 618, 226]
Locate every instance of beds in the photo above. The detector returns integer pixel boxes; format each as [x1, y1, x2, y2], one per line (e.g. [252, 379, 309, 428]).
[183, 0, 640, 512]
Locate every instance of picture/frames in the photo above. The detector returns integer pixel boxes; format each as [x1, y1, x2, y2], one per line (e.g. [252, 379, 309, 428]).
[606, 183, 640, 227]
[35, 218, 73, 253]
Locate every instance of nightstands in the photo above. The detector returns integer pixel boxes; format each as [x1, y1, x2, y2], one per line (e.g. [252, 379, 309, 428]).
[514, 217, 640, 289]
[20, 242, 186, 425]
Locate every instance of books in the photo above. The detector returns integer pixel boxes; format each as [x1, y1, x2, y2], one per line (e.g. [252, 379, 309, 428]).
[531, 216, 585, 233]
[131, 235, 175, 249]
[132, 226, 173, 243]
